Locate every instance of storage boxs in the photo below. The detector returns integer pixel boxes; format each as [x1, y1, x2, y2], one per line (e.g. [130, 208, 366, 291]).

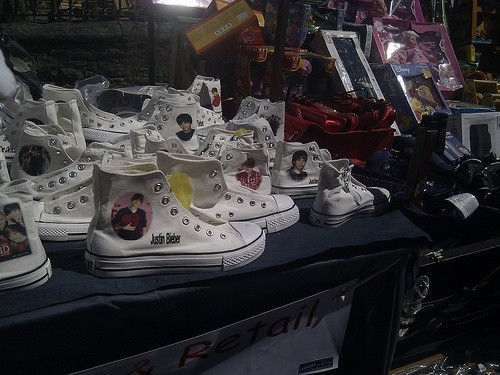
[185, 0, 500, 157]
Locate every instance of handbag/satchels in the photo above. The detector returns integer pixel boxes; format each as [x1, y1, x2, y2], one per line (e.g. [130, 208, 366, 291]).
[352, 112, 484, 221]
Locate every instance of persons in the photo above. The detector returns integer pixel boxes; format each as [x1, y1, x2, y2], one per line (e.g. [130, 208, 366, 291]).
[391, 29, 440, 82]
[113, 193, 150, 241]
[360, 0, 401, 27]
[288, 150, 310, 181]
[210, 88, 221, 107]
[0, 203, 32, 263]
[18, 145, 51, 174]
[237, 157, 262, 190]
[173, 113, 196, 141]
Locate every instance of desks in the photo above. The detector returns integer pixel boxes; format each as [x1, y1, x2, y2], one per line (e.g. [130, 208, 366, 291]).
[0, 196, 434, 374]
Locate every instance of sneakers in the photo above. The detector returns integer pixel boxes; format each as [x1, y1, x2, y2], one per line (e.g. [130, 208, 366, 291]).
[53, 101, 86, 151]
[243, 140, 332, 183]
[110, 99, 199, 153]
[1, 99, 58, 158]
[27, 142, 130, 201]
[144, 132, 192, 158]
[197, 96, 285, 147]
[190, 127, 255, 161]
[83, 89, 188, 143]
[87, 160, 265, 271]
[10, 119, 83, 178]
[36, 176, 95, 242]
[155, 149, 300, 233]
[129, 124, 165, 160]
[0, 177, 55, 294]
[229, 115, 277, 167]
[311, 157, 391, 230]
[213, 141, 271, 195]
[40, 84, 122, 127]
[187, 74, 223, 116]
